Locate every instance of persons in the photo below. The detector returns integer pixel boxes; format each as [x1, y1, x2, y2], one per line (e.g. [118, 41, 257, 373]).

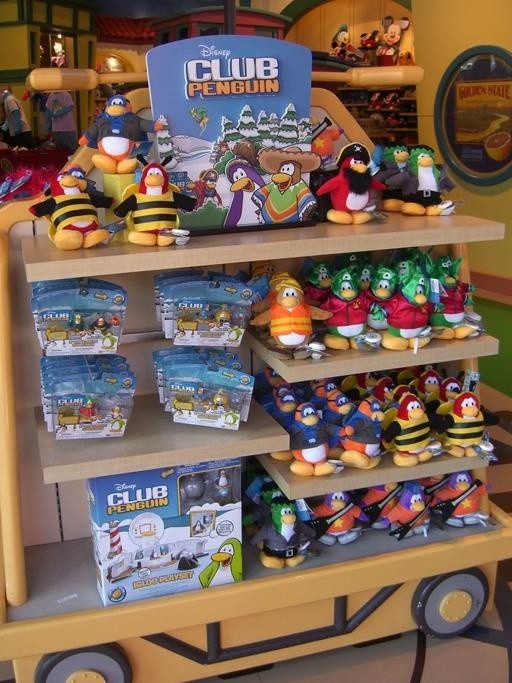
[44, 89, 78, 149]
[0, 87, 34, 147]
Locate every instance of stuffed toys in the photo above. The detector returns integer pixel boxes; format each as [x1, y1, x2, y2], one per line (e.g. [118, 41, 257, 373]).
[330, 14, 415, 66]
[29, 94, 501, 570]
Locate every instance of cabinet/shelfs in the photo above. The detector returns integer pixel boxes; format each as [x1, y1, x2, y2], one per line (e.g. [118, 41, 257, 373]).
[0, 84, 512, 682]
